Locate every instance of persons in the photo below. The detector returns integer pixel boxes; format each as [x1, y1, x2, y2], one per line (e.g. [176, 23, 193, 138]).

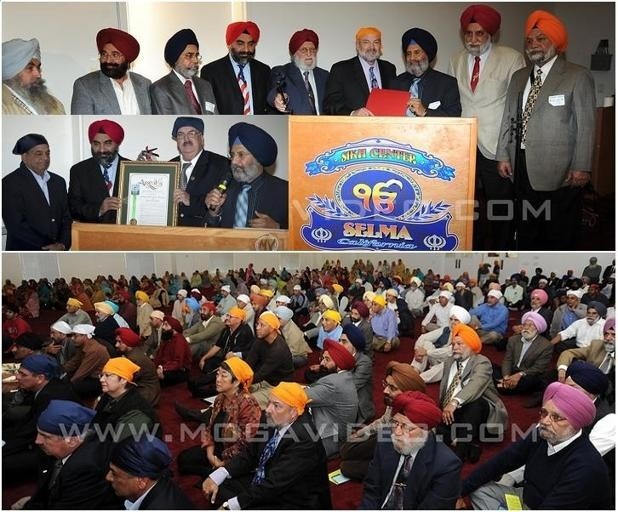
[3, 256, 615, 510]
[2, 38, 67, 115]
[133, 117, 231, 228]
[322, 26, 399, 116]
[204, 122, 289, 230]
[495, 8, 597, 251]
[199, 20, 272, 115]
[447, 3, 527, 251]
[68, 119, 133, 224]
[2, 133, 73, 251]
[148, 27, 219, 115]
[388, 26, 462, 117]
[70, 26, 155, 115]
[264, 27, 329, 115]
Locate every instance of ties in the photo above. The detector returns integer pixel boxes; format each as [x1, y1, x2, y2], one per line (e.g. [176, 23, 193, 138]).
[369, 67, 380, 90]
[251, 431, 280, 486]
[48, 459, 64, 491]
[599, 354, 611, 374]
[392, 454, 412, 509]
[236, 64, 250, 114]
[470, 56, 481, 92]
[303, 71, 316, 115]
[184, 80, 201, 115]
[232, 184, 252, 228]
[405, 78, 421, 117]
[442, 367, 458, 410]
[178, 162, 192, 205]
[520, 69, 542, 146]
[102, 164, 113, 193]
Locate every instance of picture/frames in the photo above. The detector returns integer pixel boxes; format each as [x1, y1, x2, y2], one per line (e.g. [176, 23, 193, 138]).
[116, 160, 181, 227]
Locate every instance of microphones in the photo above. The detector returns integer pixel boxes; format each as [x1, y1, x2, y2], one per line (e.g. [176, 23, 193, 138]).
[209, 169, 234, 212]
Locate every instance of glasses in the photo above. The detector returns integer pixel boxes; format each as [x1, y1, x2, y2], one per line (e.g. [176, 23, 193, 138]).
[227, 151, 252, 159]
[178, 53, 202, 59]
[382, 380, 399, 392]
[538, 410, 567, 423]
[389, 419, 418, 433]
[176, 131, 201, 138]
[98, 51, 123, 60]
[318, 356, 333, 363]
[98, 373, 116, 380]
